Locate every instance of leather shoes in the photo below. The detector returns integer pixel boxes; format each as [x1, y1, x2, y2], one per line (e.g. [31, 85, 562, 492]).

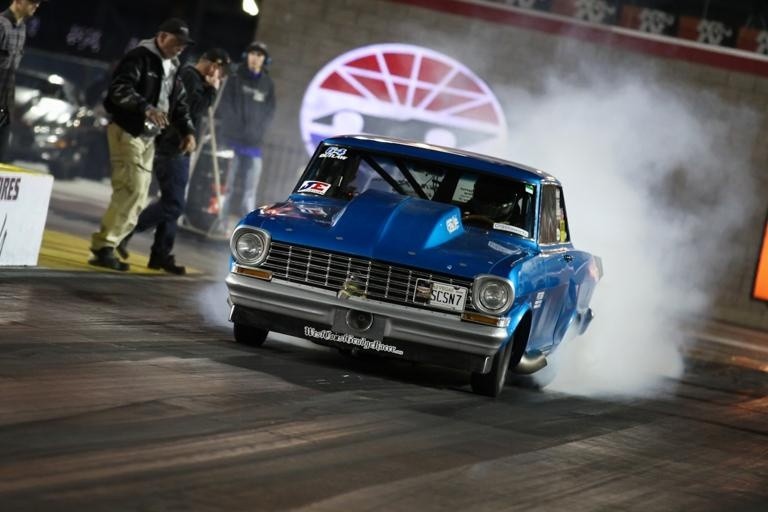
[88, 240, 186, 274]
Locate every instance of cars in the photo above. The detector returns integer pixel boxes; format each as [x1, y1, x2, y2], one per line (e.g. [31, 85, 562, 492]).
[6, 69, 110, 189]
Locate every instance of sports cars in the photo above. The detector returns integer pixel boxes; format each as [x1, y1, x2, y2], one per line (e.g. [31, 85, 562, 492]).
[222, 132, 604, 400]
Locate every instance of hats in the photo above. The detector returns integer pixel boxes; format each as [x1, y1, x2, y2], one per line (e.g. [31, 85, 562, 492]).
[201, 46, 230, 67]
[246, 41, 268, 57]
[159, 17, 194, 44]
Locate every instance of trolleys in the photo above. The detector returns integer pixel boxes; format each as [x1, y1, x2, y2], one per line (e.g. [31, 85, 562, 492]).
[177, 133, 241, 243]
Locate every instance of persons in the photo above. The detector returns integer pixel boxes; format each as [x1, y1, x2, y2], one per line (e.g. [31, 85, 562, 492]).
[218, 40, 277, 225]
[116, 49, 238, 276]
[0, 1, 40, 166]
[85, 19, 196, 271]
